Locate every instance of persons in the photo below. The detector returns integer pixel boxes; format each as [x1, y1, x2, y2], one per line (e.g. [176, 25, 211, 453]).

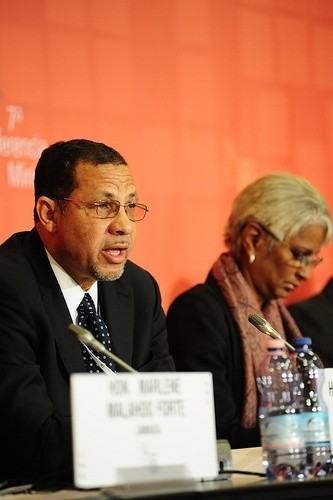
[164, 174, 333, 449]
[0, 139, 178, 490]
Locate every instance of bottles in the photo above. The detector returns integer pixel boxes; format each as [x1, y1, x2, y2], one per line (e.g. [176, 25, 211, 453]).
[295, 338, 331, 475]
[256, 340, 308, 481]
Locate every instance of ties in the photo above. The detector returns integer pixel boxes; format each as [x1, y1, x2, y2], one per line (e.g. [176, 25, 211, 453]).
[76, 293, 116, 374]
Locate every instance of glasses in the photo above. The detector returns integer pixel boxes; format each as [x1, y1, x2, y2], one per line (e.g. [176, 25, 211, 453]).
[240, 221, 324, 269]
[65, 198, 149, 222]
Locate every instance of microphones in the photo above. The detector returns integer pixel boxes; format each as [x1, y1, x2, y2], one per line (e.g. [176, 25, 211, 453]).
[248, 313, 295, 354]
[67, 325, 233, 481]
